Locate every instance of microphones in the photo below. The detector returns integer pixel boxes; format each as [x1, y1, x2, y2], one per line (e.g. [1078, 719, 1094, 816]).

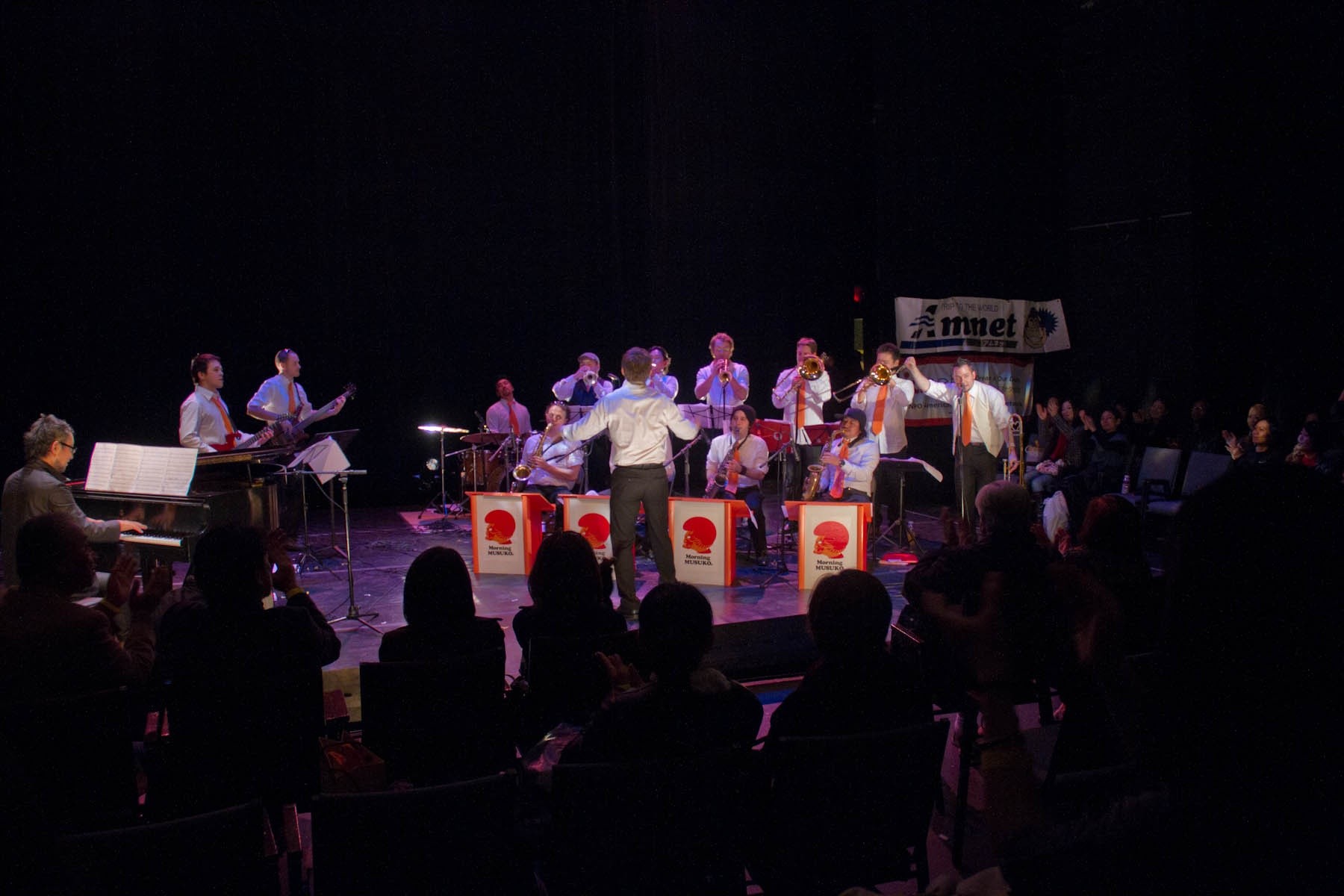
[474, 410, 484, 426]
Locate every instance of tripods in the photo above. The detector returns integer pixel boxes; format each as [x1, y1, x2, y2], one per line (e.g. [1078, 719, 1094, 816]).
[322, 478, 386, 635]
[278, 474, 324, 581]
[309, 476, 351, 560]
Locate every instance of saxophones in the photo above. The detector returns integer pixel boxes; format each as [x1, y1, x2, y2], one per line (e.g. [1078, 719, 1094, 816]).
[706, 426, 739, 499]
[802, 429, 844, 501]
[512, 423, 552, 491]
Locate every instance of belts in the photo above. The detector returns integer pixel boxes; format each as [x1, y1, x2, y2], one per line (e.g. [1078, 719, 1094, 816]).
[626, 462, 663, 469]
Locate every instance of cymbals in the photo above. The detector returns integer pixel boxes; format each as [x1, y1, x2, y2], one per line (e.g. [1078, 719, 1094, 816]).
[418, 425, 469, 433]
[461, 432, 509, 442]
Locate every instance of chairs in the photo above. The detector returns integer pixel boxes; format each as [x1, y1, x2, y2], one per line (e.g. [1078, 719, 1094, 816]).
[1, 790, 289, 896]
[529, 738, 752, 895]
[729, 718, 953, 895]
[281, 768, 535, 896]
[1028, 439, 1342, 896]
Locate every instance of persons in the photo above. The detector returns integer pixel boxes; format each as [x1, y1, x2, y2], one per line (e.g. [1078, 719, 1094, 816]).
[815, 406, 881, 505]
[1018, 385, 1151, 483]
[642, 348, 680, 487]
[1150, 388, 1344, 896]
[180, 352, 275, 455]
[247, 348, 347, 541]
[905, 353, 1017, 546]
[519, 400, 584, 506]
[0, 486, 1148, 896]
[546, 348, 703, 623]
[486, 376, 533, 442]
[703, 404, 771, 568]
[770, 335, 832, 534]
[2, 413, 148, 589]
[692, 332, 750, 495]
[849, 342, 916, 545]
[551, 352, 615, 493]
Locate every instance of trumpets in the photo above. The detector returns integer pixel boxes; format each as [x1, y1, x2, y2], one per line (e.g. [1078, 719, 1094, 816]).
[584, 371, 598, 385]
[719, 359, 729, 383]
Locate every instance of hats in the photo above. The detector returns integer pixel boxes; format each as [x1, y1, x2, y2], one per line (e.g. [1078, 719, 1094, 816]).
[730, 404, 756, 427]
[844, 407, 866, 430]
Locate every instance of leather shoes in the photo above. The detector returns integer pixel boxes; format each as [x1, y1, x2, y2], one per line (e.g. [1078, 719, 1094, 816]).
[755, 554, 768, 566]
[615, 603, 640, 619]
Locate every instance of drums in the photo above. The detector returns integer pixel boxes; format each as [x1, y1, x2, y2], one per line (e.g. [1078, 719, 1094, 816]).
[463, 451, 500, 484]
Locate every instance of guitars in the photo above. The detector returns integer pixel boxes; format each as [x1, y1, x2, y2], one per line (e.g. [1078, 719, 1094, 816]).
[269, 382, 357, 448]
[208, 413, 294, 453]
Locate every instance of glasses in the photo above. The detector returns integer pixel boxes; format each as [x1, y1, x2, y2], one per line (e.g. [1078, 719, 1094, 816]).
[60, 441, 77, 454]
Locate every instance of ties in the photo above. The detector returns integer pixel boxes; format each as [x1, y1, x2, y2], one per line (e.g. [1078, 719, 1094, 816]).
[287, 383, 296, 420]
[209, 395, 237, 448]
[829, 439, 850, 499]
[960, 392, 973, 447]
[793, 379, 807, 431]
[869, 385, 889, 436]
[506, 401, 520, 440]
[725, 443, 741, 496]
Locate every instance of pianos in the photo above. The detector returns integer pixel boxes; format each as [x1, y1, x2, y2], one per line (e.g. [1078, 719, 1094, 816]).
[65, 447, 280, 592]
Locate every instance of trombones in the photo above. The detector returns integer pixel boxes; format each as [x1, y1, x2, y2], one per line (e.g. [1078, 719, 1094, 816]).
[1002, 412, 1024, 488]
[772, 352, 830, 401]
[834, 363, 905, 403]
